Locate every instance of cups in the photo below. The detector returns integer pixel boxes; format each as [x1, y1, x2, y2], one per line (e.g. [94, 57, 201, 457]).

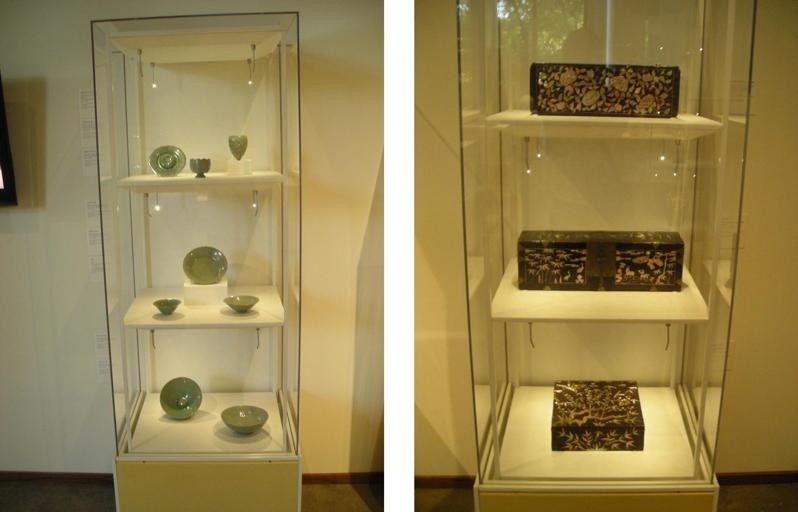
[228, 135, 248, 161]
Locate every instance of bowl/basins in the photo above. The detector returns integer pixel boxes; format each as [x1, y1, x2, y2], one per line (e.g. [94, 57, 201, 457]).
[159, 377, 203, 421]
[223, 295, 259, 313]
[153, 299, 181, 316]
[221, 406, 269, 434]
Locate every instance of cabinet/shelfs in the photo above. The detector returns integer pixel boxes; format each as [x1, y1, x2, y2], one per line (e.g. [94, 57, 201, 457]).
[454, 0, 757, 512]
[88, 11, 302, 511]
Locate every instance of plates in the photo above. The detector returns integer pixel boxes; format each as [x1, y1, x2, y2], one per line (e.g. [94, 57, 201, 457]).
[183, 247, 228, 286]
[149, 146, 186, 177]
